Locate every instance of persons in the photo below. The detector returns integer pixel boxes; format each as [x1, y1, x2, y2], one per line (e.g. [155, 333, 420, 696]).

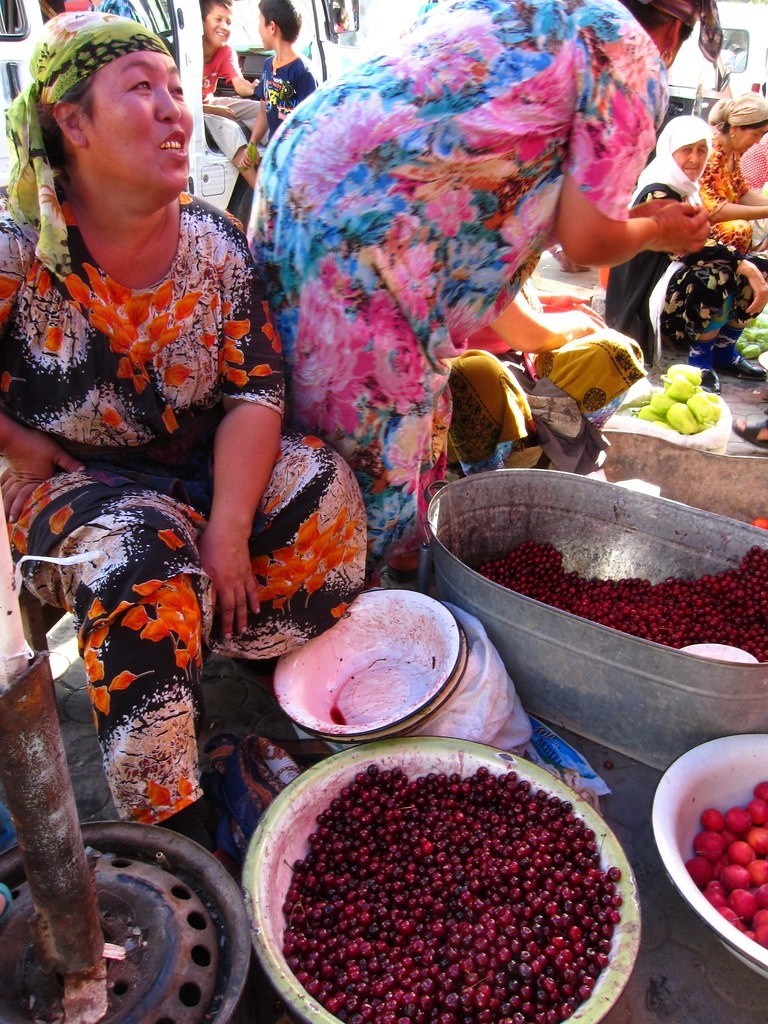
[87, 0, 154, 34]
[198, 0, 322, 190]
[605, 92, 768, 395]
[0, 11, 367, 892]
[246, 0, 710, 593]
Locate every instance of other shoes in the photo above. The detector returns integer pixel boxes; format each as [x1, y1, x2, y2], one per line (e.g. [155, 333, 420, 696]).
[714, 358, 767, 381]
[699, 368, 721, 395]
[387, 550, 436, 583]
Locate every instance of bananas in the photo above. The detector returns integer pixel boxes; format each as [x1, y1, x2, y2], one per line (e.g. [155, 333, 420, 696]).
[235, 140, 257, 171]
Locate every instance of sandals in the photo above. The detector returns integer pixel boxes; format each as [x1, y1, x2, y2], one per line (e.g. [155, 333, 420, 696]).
[732, 414, 768, 449]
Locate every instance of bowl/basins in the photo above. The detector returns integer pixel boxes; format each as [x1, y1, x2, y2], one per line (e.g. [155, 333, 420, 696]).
[273, 588, 469, 745]
[242, 736, 641, 1024]
[651, 734, 768, 979]
[680, 643, 759, 665]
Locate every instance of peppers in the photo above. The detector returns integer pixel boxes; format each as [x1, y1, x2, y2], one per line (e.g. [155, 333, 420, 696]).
[734, 311, 768, 359]
[636, 364, 725, 436]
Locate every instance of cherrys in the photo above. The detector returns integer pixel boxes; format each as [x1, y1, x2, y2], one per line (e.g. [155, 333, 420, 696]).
[683, 781, 768, 953]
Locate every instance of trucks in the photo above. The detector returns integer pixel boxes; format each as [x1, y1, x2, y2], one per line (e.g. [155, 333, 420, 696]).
[655, 4, 768, 136]
[0, 0, 380, 230]
